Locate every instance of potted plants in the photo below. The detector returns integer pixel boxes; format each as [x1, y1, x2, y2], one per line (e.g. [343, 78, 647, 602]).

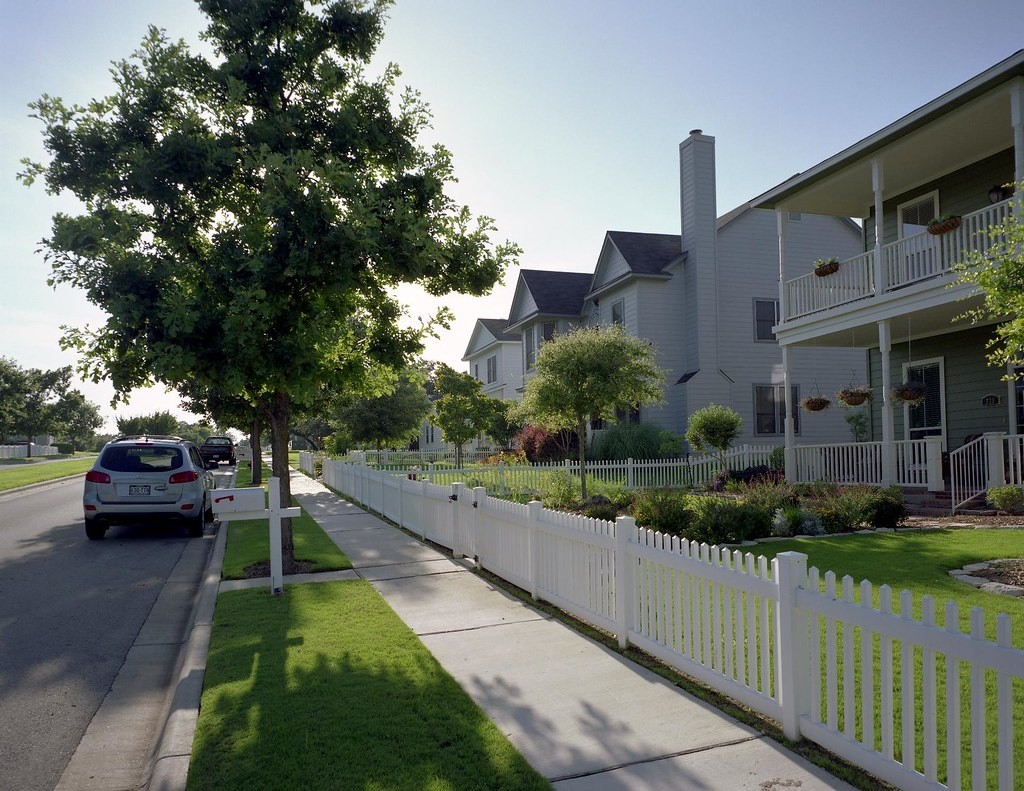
[891, 382, 928, 406]
[927, 212, 960, 235]
[835, 383, 874, 408]
[801, 394, 832, 413]
[813, 256, 839, 277]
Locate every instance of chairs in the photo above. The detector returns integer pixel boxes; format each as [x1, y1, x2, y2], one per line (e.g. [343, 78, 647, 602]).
[120, 455, 144, 473]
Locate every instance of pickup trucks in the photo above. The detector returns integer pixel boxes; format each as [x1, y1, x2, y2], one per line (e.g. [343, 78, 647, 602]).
[200, 436, 238, 466]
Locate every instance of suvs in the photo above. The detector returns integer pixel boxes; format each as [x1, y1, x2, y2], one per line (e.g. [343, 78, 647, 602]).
[83, 435, 216, 541]
